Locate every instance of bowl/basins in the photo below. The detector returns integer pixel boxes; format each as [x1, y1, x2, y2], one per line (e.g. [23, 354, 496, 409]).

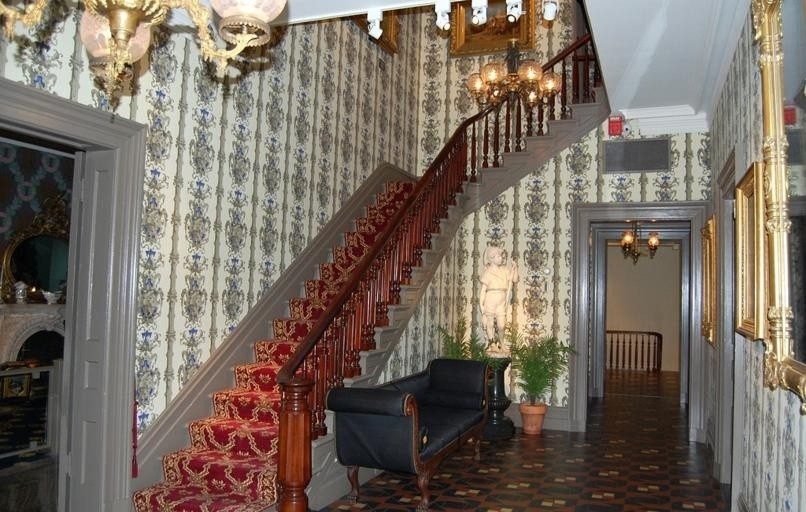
[41, 290, 64, 305]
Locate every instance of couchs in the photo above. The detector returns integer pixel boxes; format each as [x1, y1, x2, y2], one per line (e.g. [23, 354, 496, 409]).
[326, 358, 493, 511]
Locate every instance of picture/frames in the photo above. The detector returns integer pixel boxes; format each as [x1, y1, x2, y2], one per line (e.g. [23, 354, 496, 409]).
[448, 2, 535, 57]
[699, 215, 717, 349]
[732, 160, 770, 343]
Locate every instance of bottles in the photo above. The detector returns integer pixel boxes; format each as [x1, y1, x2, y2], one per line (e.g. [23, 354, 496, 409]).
[16, 288, 27, 298]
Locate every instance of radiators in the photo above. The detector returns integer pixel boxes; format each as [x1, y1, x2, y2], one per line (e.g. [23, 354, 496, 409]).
[605, 333, 658, 372]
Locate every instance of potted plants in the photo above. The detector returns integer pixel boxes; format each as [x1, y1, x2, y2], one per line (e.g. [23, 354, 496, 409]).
[510, 330, 575, 435]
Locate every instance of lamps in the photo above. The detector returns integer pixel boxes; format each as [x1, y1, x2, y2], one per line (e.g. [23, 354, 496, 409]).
[620, 221, 659, 265]
[360, 1, 560, 37]
[0, 0, 288, 112]
[465, 44, 561, 113]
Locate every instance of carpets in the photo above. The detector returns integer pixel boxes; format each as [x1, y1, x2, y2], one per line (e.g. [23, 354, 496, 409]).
[131, 178, 419, 511]
